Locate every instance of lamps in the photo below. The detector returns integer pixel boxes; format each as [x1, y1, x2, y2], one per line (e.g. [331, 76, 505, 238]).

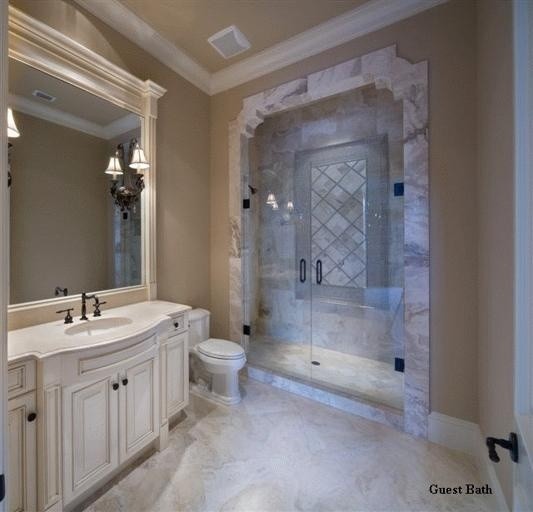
[104, 157, 124, 205]
[8, 107, 20, 188]
[117, 143, 151, 211]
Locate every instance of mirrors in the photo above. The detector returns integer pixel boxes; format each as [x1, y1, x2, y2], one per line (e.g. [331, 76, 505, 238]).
[6, 6, 167, 314]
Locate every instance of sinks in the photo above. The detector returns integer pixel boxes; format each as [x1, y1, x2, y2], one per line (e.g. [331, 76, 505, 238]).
[64, 317, 132, 337]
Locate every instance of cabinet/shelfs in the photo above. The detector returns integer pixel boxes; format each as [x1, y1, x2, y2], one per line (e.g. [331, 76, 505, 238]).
[7, 311, 190, 512]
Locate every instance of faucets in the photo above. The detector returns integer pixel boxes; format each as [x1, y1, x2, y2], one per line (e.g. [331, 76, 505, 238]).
[55, 287, 67, 296]
[81, 291, 98, 318]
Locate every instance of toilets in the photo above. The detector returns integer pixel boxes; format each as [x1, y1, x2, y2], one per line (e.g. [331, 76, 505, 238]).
[188, 308, 246, 407]
